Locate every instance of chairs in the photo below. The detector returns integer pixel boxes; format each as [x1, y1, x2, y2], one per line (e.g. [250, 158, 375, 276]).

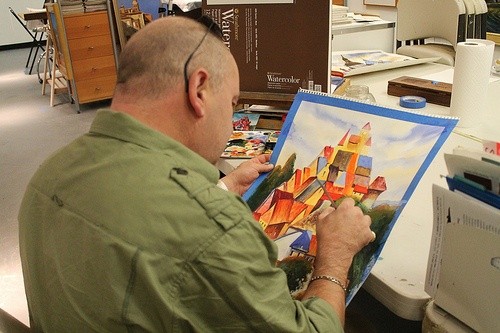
[8, 5, 70, 107]
[396, 0, 489, 67]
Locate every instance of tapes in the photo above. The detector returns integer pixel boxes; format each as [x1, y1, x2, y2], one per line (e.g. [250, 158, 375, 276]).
[400, 95, 426, 109]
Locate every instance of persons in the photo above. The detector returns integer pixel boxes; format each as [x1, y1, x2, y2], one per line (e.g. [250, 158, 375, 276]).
[17, 15, 376, 333]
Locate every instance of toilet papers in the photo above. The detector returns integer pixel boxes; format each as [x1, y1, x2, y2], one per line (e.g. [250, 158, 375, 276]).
[450, 38, 496, 128]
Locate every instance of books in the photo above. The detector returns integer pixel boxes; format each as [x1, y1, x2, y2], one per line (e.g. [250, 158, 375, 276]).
[60, 0, 108, 14]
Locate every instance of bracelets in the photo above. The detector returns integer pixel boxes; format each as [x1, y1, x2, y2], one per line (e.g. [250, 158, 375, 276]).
[307, 275, 349, 297]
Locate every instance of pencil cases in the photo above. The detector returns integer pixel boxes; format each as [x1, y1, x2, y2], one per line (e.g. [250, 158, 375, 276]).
[387, 76, 453, 107]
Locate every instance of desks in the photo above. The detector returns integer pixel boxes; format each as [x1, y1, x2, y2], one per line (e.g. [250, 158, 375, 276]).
[216, 52, 500, 332]
[331, 14, 395, 55]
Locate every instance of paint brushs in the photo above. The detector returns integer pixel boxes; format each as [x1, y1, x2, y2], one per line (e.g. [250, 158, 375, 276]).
[315, 177, 338, 210]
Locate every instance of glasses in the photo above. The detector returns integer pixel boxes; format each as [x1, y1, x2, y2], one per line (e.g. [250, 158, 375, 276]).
[184, 15, 223, 94]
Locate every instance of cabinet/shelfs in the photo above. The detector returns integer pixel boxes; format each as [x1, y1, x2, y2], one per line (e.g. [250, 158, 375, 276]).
[46, 0, 118, 114]
